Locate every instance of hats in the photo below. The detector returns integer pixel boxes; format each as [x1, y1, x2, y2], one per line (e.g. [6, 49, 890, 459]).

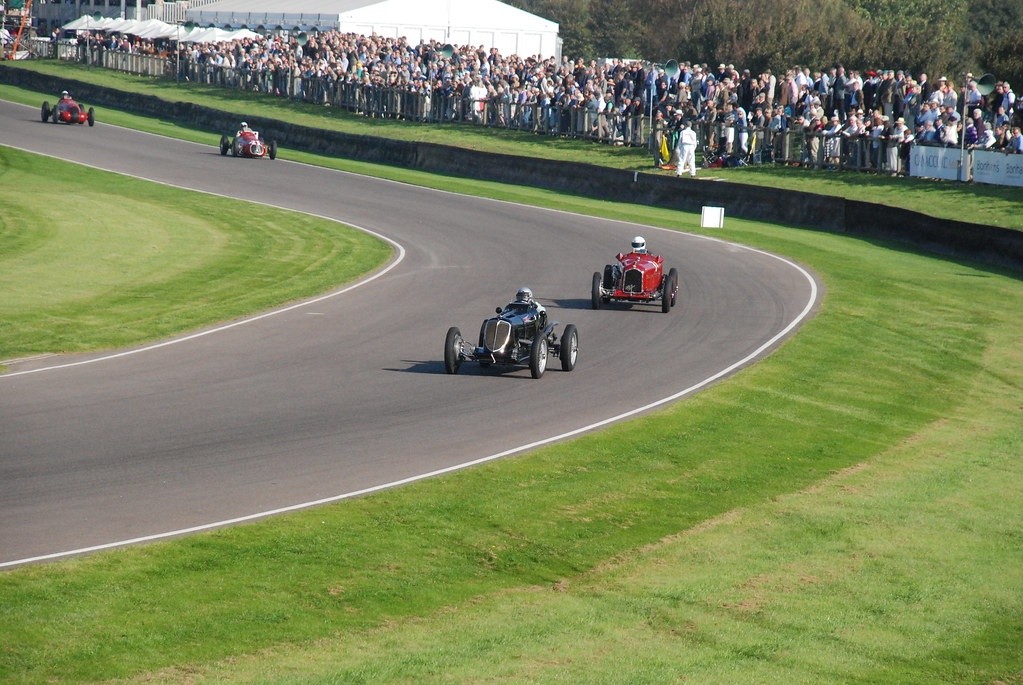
[866, 70, 875, 76]
[856, 109, 865, 115]
[895, 118, 906, 123]
[673, 110, 683, 116]
[938, 76, 947, 82]
[717, 64, 725, 69]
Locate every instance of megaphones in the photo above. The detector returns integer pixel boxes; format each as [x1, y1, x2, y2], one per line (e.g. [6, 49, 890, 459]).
[435, 44, 453, 58]
[180, 21, 194, 33]
[653, 59, 678, 77]
[89, 11, 101, 21]
[292, 32, 307, 45]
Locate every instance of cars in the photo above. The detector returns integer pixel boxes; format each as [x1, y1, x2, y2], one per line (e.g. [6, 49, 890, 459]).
[41, 101, 95, 126]
[445, 300, 578, 380]
[591, 251, 678, 313]
[220, 134, 277, 158]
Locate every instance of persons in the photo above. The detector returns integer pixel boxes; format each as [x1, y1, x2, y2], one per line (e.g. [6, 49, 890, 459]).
[62, 90, 71, 99]
[515, 287, 546, 318]
[50, 28, 59, 41]
[81, 29, 1023, 176]
[631, 236, 646, 251]
[236, 121, 250, 136]
[676, 118, 697, 178]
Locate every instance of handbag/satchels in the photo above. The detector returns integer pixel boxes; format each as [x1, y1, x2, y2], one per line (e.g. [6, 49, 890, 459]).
[1007, 92, 1022, 112]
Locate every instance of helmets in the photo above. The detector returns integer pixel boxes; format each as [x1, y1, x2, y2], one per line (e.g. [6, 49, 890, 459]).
[516, 287, 532, 303]
[631, 236, 646, 253]
[240, 122, 247, 130]
[62, 91, 69, 97]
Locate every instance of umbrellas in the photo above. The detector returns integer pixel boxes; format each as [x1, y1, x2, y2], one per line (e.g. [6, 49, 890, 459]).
[61, 15, 263, 44]
